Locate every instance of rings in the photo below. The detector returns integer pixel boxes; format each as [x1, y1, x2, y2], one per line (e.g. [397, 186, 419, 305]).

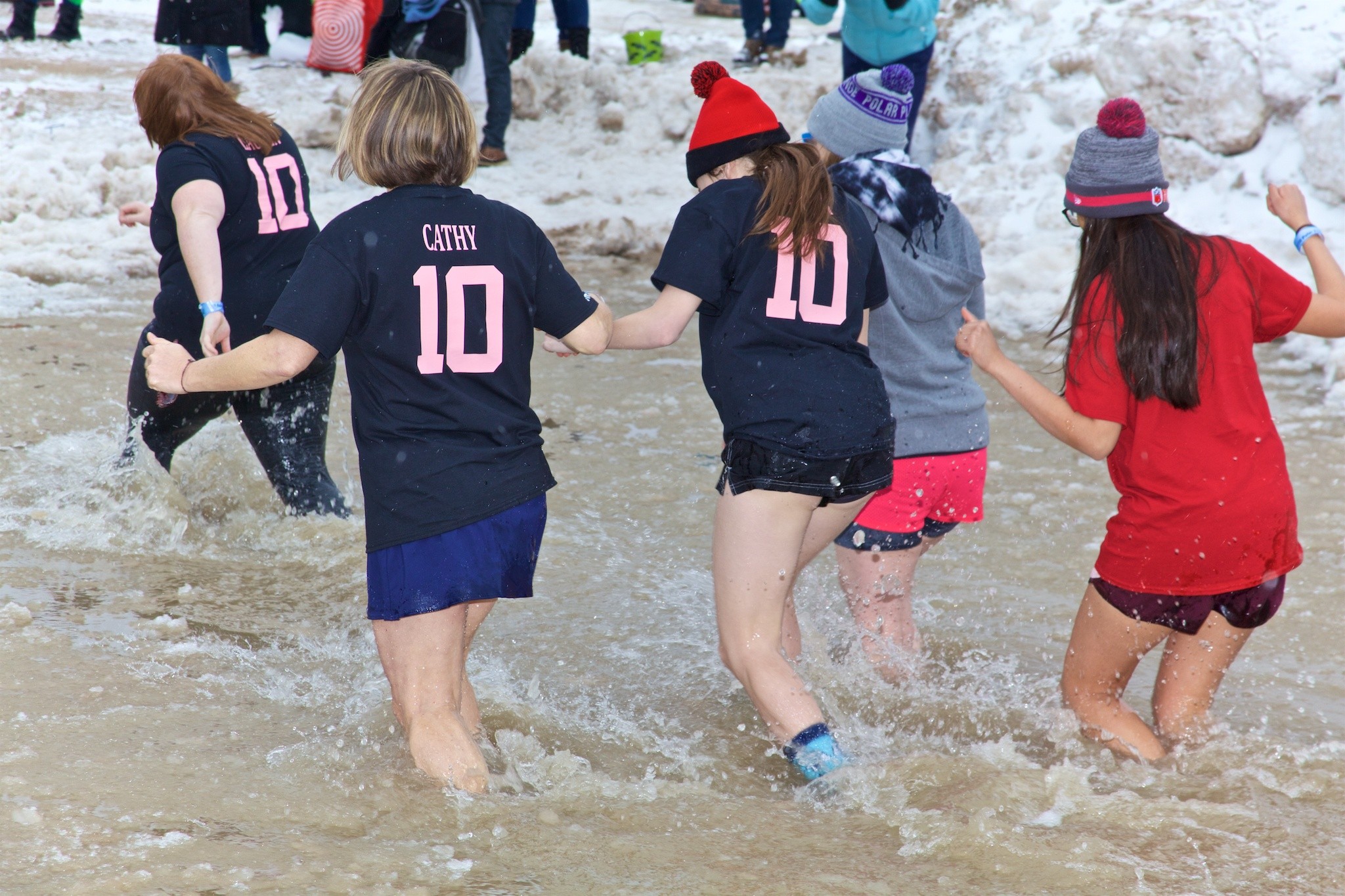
[964, 336, 967, 342]
[959, 328, 962, 333]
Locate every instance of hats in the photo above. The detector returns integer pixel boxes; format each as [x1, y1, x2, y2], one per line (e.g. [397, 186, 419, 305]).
[1063, 97, 1169, 217]
[808, 64, 914, 159]
[686, 61, 790, 187]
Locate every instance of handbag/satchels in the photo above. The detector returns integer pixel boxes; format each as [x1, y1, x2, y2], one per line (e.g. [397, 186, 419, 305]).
[306, 0, 383, 73]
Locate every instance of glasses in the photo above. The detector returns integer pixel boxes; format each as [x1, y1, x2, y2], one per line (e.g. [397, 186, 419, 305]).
[1062, 208, 1081, 227]
[801, 131, 814, 144]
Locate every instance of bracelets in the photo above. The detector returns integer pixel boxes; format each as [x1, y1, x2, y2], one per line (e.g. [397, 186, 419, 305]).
[199, 302, 224, 317]
[1294, 224, 1324, 254]
[180, 359, 196, 393]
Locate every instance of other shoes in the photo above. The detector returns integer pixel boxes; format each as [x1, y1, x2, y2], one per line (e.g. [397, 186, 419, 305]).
[477, 145, 504, 167]
[757, 44, 781, 64]
[733, 40, 762, 63]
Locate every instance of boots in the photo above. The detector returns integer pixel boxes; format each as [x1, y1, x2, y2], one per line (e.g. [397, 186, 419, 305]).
[559, 29, 589, 60]
[38, 0, 82, 40]
[507, 28, 534, 64]
[0, 0, 38, 42]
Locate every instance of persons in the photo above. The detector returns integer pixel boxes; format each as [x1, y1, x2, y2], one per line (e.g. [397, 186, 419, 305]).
[801, 65, 989, 667]
[953, 98, 1345, 760]
[154, 0, 250, 80]
[543, 62, 893, 785]
[142, 57, 614, 795]
[6, 0, 83, 42]
[799, 0, 939, 154]
[734, 0, 795, 64]
[118, 53, 353, 518]
[470, 0, 512, 166]
[505, 0, 589, 60]
